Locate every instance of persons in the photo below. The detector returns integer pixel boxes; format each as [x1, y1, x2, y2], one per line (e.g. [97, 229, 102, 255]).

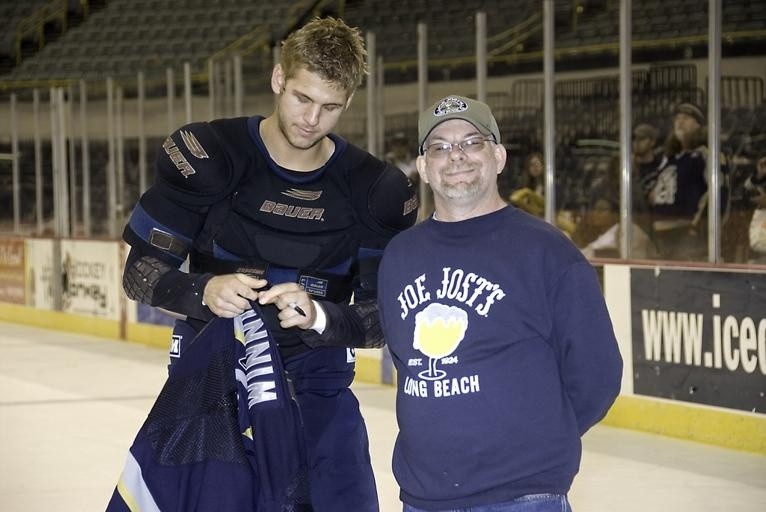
[377, 93, 625, 510]
[119, 16, 420, 511]
[53, 103, 766, 265]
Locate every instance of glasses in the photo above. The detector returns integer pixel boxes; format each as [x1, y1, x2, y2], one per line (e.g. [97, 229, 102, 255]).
[421, 136, 496, 159]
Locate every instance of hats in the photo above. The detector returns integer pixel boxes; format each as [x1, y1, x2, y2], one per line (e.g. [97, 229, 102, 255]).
[416, 93, 503, 157]
